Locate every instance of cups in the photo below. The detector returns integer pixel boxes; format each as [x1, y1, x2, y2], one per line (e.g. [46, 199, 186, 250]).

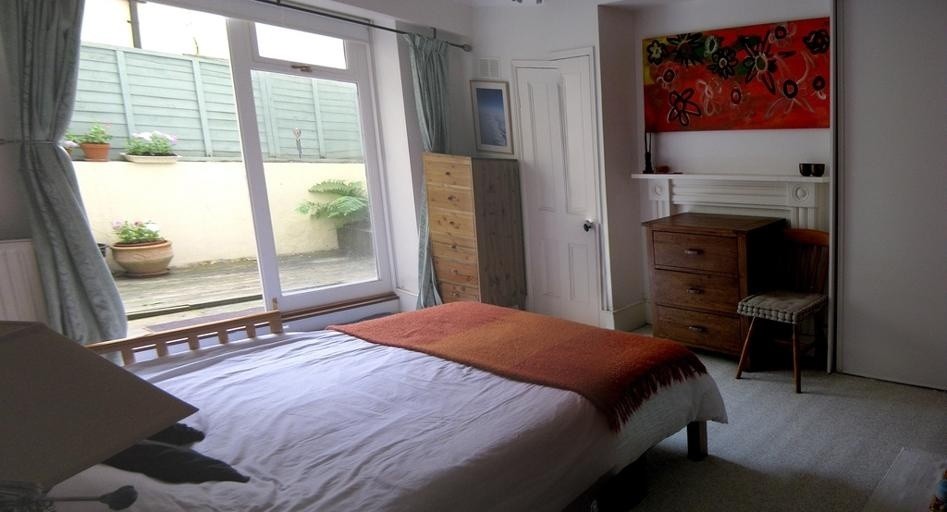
[655, 165, 669, 173]
[799, 162, 826, 178]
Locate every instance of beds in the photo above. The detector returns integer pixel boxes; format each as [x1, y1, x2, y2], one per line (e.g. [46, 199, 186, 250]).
[0, 302, 710, 510]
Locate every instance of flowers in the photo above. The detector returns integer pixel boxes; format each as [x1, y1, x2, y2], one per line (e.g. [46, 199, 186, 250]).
[109, 218, 173, 244]
[127, 129, 177, 153]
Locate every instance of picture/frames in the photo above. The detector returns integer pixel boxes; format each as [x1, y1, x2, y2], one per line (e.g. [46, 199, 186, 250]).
[468, 79, 514, 157]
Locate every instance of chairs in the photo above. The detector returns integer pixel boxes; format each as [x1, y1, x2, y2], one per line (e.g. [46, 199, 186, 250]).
[736, 229, 828, 394]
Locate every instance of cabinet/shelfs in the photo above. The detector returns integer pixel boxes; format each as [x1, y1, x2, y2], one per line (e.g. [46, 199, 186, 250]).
[422, 152, 526, 312]
[645, 220, 790, 373]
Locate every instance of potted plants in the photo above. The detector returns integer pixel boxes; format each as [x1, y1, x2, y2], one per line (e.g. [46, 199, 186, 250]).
[297, 178, 373, 257]
[79, 124, 113, 162]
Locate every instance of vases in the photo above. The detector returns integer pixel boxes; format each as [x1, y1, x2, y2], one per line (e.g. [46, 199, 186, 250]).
[107, 240, 173, 277]
[121, 153, 183, 163]
